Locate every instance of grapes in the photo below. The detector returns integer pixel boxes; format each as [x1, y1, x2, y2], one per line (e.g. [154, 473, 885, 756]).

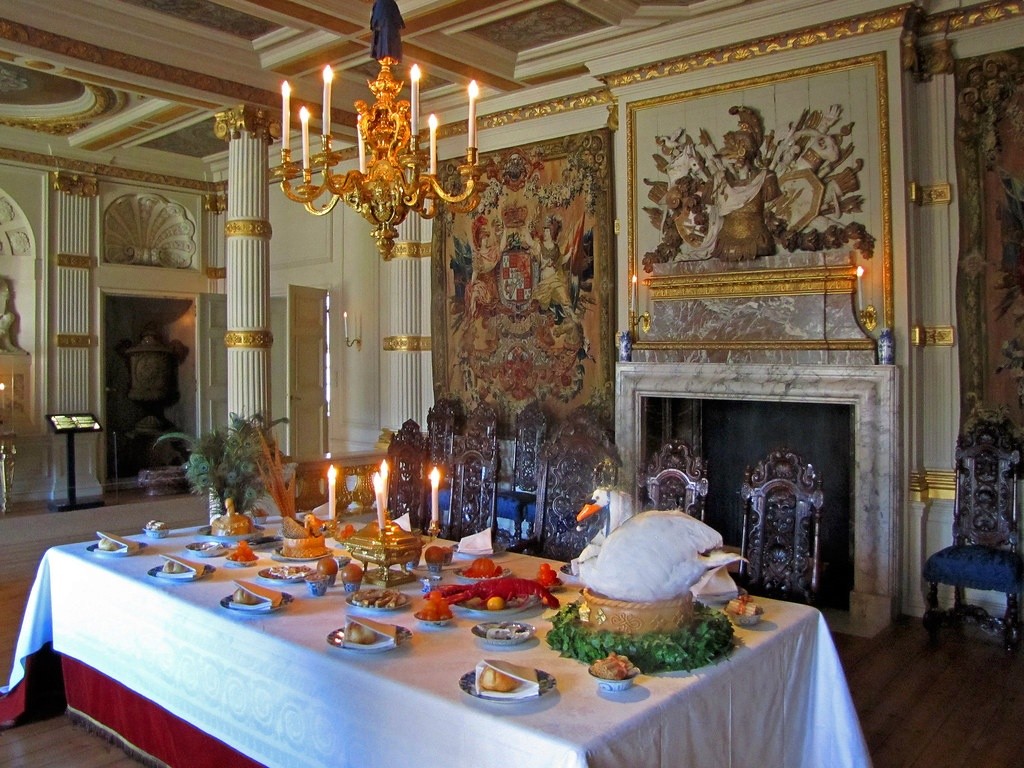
[414, 590, 454, 621]
[230, 540, 256, 562]
[533, 564, 561, 585]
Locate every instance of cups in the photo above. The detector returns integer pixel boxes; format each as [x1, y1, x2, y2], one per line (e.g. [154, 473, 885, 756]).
[407, 551, 453, 596]
[304, 556, 361, 597]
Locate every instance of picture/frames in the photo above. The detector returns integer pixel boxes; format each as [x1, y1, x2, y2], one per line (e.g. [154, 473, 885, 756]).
[956, 51, 1024, 475]
[430, 126, 615, 446]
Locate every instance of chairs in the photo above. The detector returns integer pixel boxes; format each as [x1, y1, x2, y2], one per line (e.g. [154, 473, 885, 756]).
[383, 394, 826, 611]
[921, 398, 1024, 657]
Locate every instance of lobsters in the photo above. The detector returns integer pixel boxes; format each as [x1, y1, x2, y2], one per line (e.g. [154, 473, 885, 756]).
[425, 579, 559, 610]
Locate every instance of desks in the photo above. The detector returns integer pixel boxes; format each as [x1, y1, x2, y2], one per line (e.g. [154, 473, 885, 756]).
[0, 513, 873, 768]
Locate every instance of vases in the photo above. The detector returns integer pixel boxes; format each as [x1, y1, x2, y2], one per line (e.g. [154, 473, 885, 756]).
[878, 326, 896, 365]
[619, 330, 632, 362]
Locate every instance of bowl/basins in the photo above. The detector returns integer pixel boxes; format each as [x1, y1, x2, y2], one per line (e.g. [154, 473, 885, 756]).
[210, 500, 326, 558]
[732, 614, 763, 627]
[583, 588, 692, 636]
[588, 667, 640, 693]
[141, 526, 171, 539]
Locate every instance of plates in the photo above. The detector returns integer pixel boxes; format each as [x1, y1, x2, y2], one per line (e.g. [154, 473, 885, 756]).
[345, 588, 456, 629]
[458, 669, 557, 705]
[147, 564, 217, 581]
[326, 624, 414, 654]
[257, 565, 317, 584]
[450, 593, 536, 618]
[87, 542, 148, 557]
[198, 524, 347, 561]
[186, 541, 232, 558]
[226, 555, 260, 566]
[450, 543, 581, 593]
[471, 622, 536, 647]
[220, 592, 294, 616]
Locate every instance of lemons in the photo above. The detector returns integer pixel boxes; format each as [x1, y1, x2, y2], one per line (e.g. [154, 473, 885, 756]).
[487, 596, 506, 610]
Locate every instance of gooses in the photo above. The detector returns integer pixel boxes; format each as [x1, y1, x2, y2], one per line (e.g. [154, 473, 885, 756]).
[578, 487, 741, 604]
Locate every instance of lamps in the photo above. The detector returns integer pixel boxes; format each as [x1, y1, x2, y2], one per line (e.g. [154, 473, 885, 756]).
[630, 275, 652, 334]
[275, 0, 488, 263]
[856, 265, 878, 332]
[317, 458, 441, 588]
[343, 312, 361, 352]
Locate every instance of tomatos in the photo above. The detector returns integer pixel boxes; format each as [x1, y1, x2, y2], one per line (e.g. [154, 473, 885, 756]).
[341, 564, 363, 581]
[316, 557, 338, 574]
[425, 546, 444, 562]
